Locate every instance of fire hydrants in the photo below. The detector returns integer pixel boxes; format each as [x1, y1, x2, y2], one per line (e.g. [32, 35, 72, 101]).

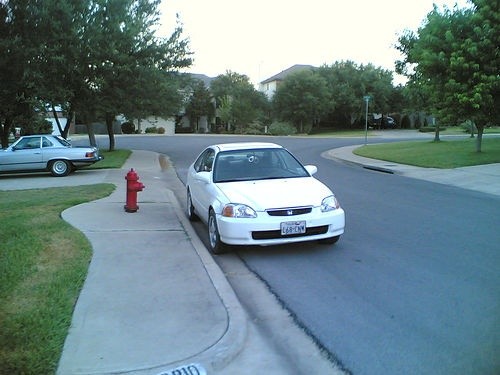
[124, 168, 145, 213]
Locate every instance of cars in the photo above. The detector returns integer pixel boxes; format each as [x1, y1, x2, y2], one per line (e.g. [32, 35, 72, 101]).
[186, 143, 345, 253]
[0, 135, 104, 177]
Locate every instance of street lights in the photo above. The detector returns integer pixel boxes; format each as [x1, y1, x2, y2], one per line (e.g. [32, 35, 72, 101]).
[364, 95, 370, 142]
